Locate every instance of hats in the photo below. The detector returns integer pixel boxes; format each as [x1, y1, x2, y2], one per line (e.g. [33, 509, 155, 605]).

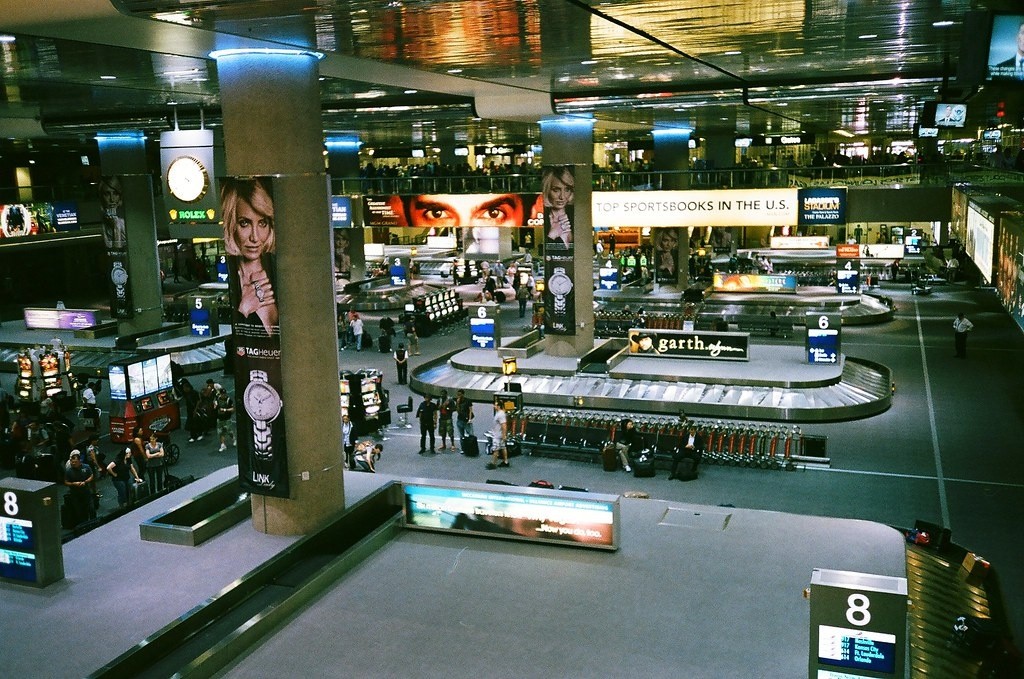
[220, 389, 227, 394]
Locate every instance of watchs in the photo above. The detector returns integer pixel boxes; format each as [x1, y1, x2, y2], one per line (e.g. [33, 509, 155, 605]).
[111, 261, 128, 302]
[547, 267, 573, 318]
[244, 370, 283, 466]
[254, 282, 264, 303]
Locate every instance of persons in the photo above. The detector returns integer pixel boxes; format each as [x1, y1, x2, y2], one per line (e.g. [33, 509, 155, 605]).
[594, 225, 966, 287]
[668, 426, 703, 480]
[622, 305, 646, 328]
[996, 22, 1024, 67]
[953, 313, 974, 359]
[615, 419, 639, 472]
[543, 166, 574, 249]
[768, 311, 780, 336]
[221, 178, 278, 337]
[711, 318, 728, 333]
[358, 146, 1024, 188]
[938, 105, 955, 127]
[333, 228, 535, 384]
[341, 390, 509, 472]
[172, 264, 182, 283]
[99, 177, 127, 251]
[397, 195, 544, 228]
[0, 369, 237, 525]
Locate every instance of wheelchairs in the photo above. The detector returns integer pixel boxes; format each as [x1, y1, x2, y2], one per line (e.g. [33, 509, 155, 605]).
[153, 430, 180, 466]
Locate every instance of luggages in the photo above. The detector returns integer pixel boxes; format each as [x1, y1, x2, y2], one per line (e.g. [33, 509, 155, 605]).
[378, 329, 389, 352]
[60, 491, 76, 530]
[460, 435, 479, 456]
[362, 330, 373, 348]
[164, 469, 181, 488]
[129, 481, 149, 503]
[602, 443, 616, 472]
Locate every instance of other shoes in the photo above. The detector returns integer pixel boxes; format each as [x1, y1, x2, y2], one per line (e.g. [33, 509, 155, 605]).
[347, 468, 354, 471]
[461, 450, 464, 454]
[623, 465, 631, 472]
[451, 445, 456, 452]
[219, 446, 227, 452]
[497, 461, 510, 467]
[188, 438, 195, 442]
[430, 448, 435, 454]
[419, 449, 425, 454]
[232, 439, 236, 446]
[668, 474, 675, 480]
[344, 460, 349, 468]
[437, 446, 446, 451]
[484, 462, 497, 469]
[197, 435, 204, 440]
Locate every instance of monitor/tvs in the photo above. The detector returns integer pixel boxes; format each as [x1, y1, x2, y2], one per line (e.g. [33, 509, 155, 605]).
[956, 10, 1024, 84]
[984, 129, 1002, 140]
[913, 101, 967, 139]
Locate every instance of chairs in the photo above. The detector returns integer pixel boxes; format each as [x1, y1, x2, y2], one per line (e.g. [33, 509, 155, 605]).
[397, 396, 413, 429]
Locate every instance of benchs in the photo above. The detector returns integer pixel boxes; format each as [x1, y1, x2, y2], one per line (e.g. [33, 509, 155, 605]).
[595, 320, 634, 337]
[520, 421, 700, 473]
[738, 314, 794, 338]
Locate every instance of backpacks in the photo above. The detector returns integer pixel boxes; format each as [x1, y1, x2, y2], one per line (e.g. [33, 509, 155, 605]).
[633, 458, 655, 478]
[676, 462, 698, 481]
[354, 440, 373, 455]
